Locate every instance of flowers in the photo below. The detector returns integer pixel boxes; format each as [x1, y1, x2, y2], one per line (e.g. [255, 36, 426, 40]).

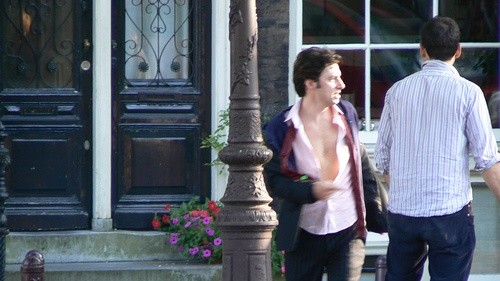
[152, 195, 286, 275]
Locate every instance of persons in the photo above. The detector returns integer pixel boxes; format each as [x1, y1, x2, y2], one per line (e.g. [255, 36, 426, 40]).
[374, 17, 500, 281]
[261, 46, 389, 281]
